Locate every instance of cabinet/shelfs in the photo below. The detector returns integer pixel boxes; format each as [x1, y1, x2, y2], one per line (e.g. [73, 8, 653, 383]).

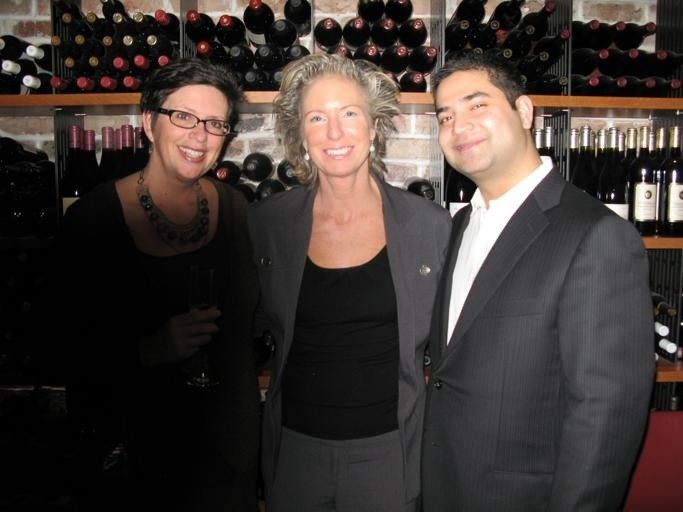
[0, 90, 683, 385]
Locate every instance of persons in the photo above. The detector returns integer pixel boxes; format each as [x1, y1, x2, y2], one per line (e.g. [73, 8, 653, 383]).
[245, 54, 454, 512]
[419, 53, 658, 512]
[63, 57, 250, 512]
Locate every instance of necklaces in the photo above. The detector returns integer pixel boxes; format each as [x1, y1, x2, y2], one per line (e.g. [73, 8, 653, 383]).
[134, 171, 210, 252]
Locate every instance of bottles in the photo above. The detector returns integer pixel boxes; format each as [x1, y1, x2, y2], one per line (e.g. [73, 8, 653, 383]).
[0, 0, 683, 97]
[61, 123, 296, 211]
[528, 124, 683, 234]
[403, 175, 434, 202]
[647, 288, 678, 410]
[0, 136, 56, 239]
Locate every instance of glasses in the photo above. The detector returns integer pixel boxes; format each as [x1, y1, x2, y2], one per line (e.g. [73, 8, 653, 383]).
[157, 105, 234, 139]
